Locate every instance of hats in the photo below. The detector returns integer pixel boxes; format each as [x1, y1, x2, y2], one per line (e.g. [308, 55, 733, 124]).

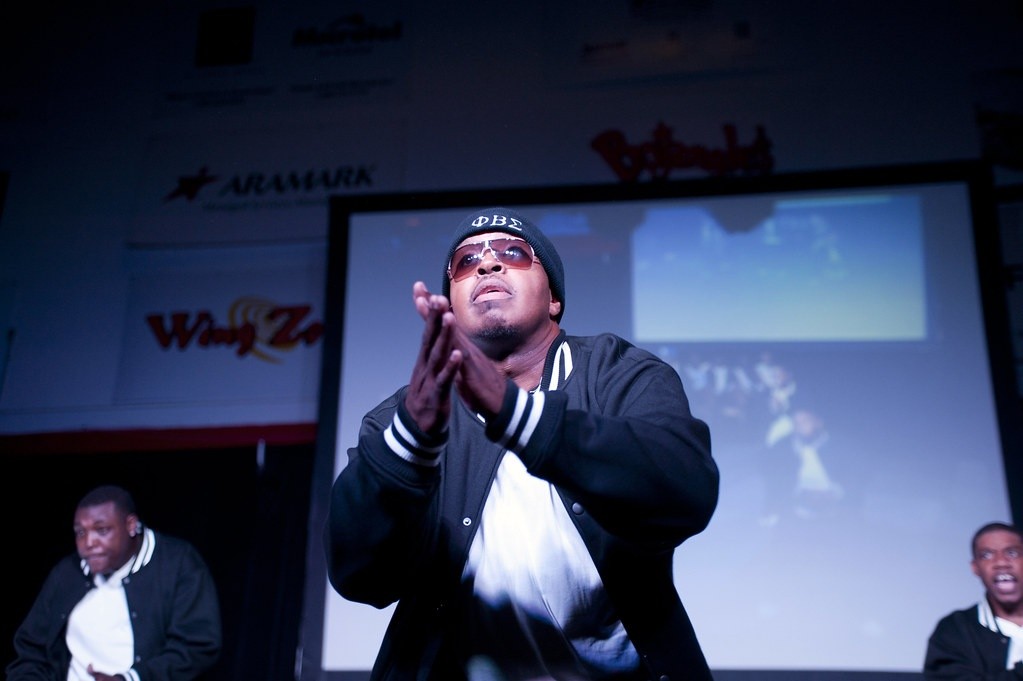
[441, 207, 565, 325]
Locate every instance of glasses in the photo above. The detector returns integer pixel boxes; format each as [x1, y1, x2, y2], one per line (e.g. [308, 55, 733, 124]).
[447, 238, 541, 282]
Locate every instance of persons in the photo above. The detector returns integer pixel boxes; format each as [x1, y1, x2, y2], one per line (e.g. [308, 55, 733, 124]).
[5, 487, 221, 681]
[923, 522, 1023, 681]
[327, 208, 719, 681]
